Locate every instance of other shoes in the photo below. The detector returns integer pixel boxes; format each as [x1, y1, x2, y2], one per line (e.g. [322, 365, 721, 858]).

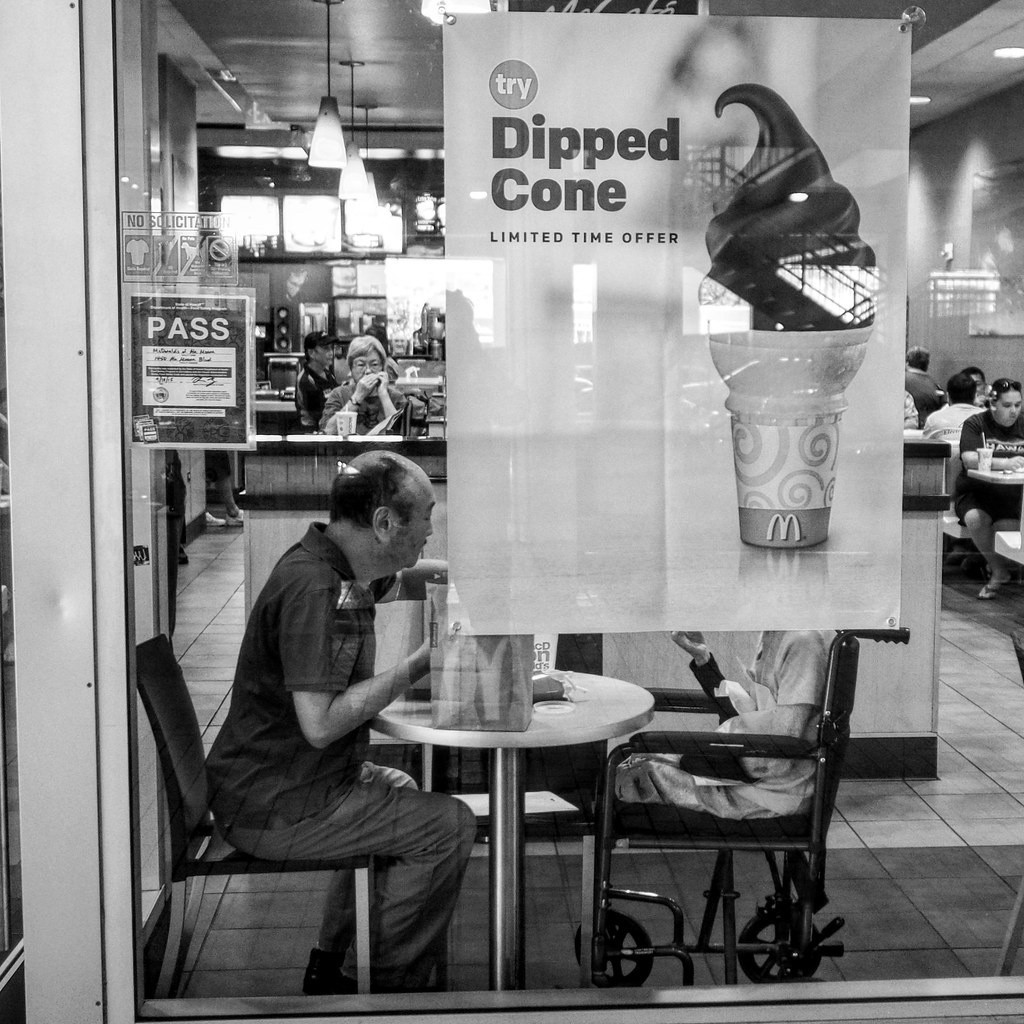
[178, 544, 188, 564]
[943, 545, 965, 563]
[225, 509, 244, 526]
[206, 511, 226, 526]
[301, 948, 446, 995]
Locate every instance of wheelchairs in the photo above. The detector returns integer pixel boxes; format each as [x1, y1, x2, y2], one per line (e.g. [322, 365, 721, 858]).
[575, 626, 912, 989]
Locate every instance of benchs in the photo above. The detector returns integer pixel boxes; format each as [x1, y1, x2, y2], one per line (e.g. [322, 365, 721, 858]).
[995, 494, 1024, 565]
[942, 442, 1021, 538]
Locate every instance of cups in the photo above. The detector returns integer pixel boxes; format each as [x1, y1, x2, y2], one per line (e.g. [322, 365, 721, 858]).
[335, 411, 358, 436]
[976, 448, 994, 474]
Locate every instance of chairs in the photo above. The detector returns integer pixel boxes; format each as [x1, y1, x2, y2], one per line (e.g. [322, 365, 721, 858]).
[136, 634, 377, 1001]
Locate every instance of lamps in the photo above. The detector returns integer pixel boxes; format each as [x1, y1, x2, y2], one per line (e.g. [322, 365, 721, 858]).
[344, 103, 382, 236]
[338, 60, 368, 201]
[309, 1, 349, 170]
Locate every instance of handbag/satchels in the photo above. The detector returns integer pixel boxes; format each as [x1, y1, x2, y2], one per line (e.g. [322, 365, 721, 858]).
[424, 575, 534, 732]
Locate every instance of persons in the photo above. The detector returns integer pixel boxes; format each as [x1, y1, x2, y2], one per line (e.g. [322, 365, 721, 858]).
[905, 391, 919, 429]
[610, 630, 828, 823]
[205, 450, 244, 527]
[959, 366, 990, 407]
[906, 346, 940, 430]
[205, 450, 475, 994]
[319, 334, 409, 435]
[954, 377, 1024, 600]
[922, 372, 985, 501]
[294, 330, 343, 435]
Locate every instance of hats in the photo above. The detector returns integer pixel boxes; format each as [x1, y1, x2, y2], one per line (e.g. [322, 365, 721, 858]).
[304, 331, 338, 349]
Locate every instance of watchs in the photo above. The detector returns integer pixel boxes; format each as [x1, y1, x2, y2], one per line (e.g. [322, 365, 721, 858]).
[350, 398, 361, 406]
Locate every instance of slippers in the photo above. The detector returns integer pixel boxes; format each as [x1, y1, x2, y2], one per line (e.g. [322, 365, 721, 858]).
[976, 571, 1010, 599]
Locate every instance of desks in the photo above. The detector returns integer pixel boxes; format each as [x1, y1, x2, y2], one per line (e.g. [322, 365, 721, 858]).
[368, 671, 653, 990]
[968, 468, 1024, 486]
